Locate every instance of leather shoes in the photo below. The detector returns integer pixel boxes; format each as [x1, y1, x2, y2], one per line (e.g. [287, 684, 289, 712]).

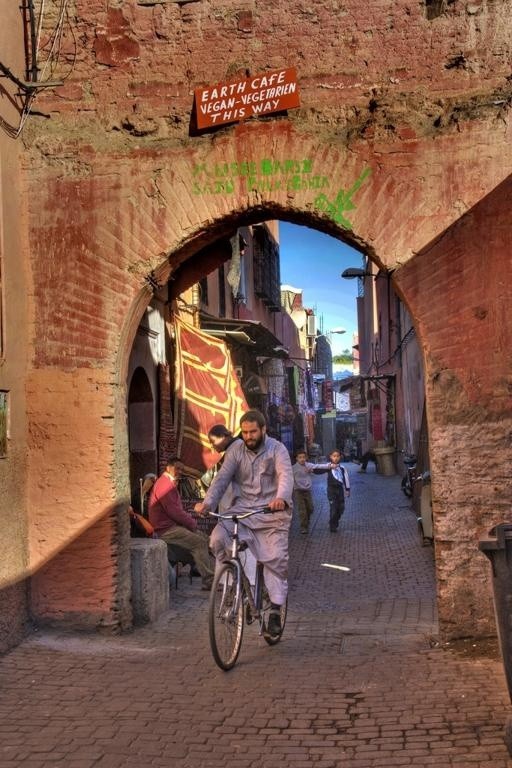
[267, 611, 283, 636]
[330, 519, 339, 531]
[226, 577, 248, 606]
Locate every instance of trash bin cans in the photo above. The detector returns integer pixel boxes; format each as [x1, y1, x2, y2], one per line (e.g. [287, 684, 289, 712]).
[478, 522, 512, 704]
[374, 447, 397, 476]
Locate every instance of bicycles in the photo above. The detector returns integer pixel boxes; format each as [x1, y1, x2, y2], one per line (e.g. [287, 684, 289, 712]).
[187, 500, 289, 671]
[394, 446, 418, 498]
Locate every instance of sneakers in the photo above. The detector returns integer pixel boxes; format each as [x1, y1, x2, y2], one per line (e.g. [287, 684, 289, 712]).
[202, 579, 223, 592]
[301, 527, 307, 533]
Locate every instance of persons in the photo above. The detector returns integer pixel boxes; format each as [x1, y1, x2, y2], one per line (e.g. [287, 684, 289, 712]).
[352, 451, 373, 473]
[293, 450, 339, 534]
[148, 456, 214, 591]
[194, 409, 293, 634]
[304, 448, 350, 532]
